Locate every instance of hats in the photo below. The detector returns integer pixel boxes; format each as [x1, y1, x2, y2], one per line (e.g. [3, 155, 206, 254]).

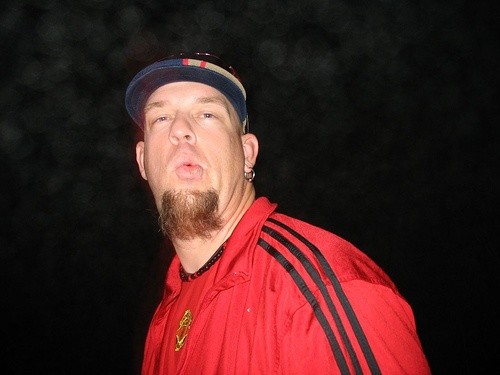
[124, 52, 247, 134]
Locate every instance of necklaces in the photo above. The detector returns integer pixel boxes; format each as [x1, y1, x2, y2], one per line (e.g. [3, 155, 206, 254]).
[178, 244, 225, 282]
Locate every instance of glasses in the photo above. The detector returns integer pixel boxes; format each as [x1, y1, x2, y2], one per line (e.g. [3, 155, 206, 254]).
[152, 51, 249, 93]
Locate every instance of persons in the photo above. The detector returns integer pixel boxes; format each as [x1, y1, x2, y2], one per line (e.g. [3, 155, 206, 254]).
[124, 50, 431, 375]
